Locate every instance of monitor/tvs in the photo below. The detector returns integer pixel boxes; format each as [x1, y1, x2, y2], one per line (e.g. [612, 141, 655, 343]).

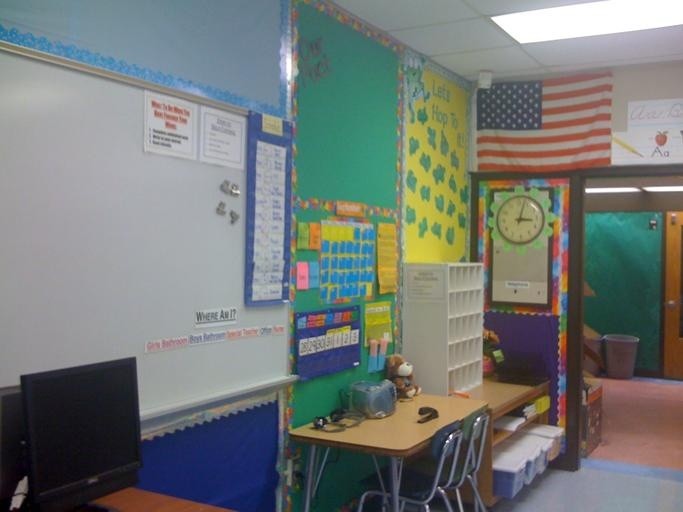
[20, 355, 144, 512]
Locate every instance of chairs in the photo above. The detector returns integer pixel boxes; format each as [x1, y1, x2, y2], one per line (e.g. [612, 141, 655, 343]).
[358, 419, 463, 512]
[412, 406, 491, 512]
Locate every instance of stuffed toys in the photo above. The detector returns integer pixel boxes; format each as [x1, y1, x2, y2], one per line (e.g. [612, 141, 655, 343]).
[384, 353, 421, 397]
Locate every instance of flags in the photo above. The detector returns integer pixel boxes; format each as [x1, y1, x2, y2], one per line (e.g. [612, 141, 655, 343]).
[475, 70, 613, 171]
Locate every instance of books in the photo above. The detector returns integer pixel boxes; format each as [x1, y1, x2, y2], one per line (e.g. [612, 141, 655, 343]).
[496, 402, 537, 432]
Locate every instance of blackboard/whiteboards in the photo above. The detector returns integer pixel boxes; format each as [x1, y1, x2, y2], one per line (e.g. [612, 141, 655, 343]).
[0, 39, 298, 429]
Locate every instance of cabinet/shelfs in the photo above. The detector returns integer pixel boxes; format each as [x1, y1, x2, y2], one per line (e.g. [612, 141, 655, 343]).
[439, 371, 548, 504]
[404, 260, 484, 395]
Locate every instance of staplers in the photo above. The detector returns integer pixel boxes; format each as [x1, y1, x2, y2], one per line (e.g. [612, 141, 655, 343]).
[417, 407, 438, 423]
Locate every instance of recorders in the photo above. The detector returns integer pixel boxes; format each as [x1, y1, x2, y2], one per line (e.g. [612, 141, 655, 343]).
[350, 378, 395, 418]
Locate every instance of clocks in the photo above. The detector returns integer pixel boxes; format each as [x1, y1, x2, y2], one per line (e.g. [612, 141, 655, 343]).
[496, 196, 545, 244]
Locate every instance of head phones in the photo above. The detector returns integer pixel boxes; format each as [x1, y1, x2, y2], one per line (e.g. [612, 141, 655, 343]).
[313, 416, 345, 432]
[330, 408, 366, 428]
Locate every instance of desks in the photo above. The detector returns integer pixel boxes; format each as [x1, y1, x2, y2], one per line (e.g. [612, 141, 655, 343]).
[291, 394, 489, 512]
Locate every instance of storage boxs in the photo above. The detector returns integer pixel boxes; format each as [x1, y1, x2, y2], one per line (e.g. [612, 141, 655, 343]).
[492, 418, 565, 499]
